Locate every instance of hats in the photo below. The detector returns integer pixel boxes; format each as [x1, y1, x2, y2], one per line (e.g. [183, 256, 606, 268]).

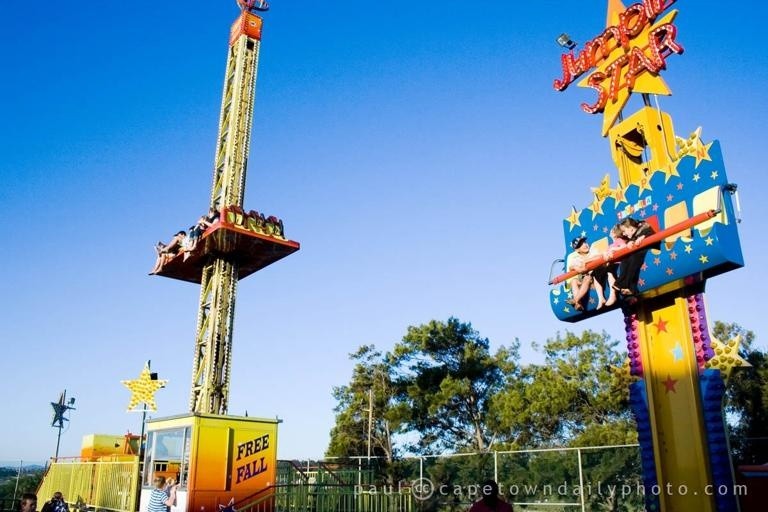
[571, 236, 586, 249]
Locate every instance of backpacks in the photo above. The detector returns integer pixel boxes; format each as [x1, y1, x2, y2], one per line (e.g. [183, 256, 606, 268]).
[55, 501, 68, 512]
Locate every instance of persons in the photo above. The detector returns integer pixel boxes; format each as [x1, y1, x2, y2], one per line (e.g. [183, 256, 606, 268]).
[18, 493, 38, 511]
[469, 477, 513, 512]
[566, 234, 597, 312]
[147, 207, 221, 275]
[611, 217, 663, 298]
[148, 474, 179, 511]
[591, 223, 629, 310]
[41, 490, 68, 511]
